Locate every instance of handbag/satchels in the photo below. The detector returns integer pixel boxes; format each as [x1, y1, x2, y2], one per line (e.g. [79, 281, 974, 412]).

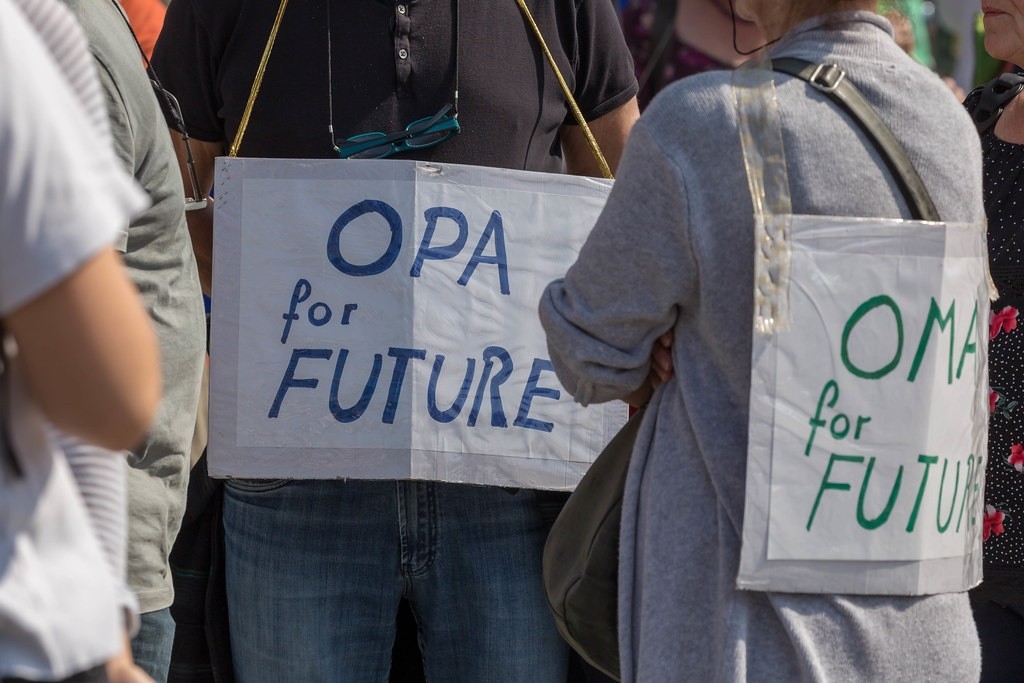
[542, 396, 648, 683]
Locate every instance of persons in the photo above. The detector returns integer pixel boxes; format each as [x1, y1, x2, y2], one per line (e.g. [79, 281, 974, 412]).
[962, 1, 1024, 682]
[534, 1, 987, 682]
[145, 0, 642, 683]
[0, 2, 240, 683]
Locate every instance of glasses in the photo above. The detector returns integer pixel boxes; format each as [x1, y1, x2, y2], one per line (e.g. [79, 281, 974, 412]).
[336, 103, 463, 159]
[149, 79, 208, 212]
